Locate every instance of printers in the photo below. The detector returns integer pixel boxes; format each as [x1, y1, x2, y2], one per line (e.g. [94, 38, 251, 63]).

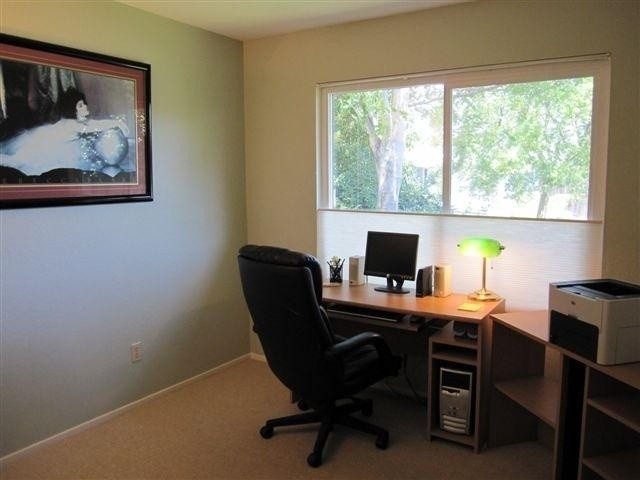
[548, 278, 640, 365]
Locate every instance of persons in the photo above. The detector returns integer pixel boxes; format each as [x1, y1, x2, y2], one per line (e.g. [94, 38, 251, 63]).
[1, 89, 130, 175]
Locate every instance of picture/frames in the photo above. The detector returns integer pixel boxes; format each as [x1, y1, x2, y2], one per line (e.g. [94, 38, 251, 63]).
[0, 29, 153, 210]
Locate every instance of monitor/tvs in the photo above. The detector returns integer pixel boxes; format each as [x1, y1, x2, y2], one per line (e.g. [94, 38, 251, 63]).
[364, 231, 419, 293]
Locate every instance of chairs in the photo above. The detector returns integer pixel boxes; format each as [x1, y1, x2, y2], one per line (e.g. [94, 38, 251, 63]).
[235, 241, 399, 470]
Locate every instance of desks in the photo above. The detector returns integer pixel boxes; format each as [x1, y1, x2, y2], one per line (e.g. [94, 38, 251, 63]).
[319, 282, 506, 455]
[489, 308, 640, 477]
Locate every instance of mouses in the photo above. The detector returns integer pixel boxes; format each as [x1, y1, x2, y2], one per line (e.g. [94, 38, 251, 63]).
[409, 315, 425, 323]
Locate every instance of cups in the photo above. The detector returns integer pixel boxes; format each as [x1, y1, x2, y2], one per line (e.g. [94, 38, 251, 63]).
[330, 265, 343, 283]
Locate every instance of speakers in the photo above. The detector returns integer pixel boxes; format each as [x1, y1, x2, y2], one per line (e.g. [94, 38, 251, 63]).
[434, 263, 453, 297]
[349, 255, 368, 285]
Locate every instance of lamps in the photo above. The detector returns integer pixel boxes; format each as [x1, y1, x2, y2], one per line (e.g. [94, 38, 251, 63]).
[457, 237, 507, 302]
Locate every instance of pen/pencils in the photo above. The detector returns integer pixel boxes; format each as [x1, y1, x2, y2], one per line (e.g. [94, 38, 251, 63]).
[326, 258, 345, 279]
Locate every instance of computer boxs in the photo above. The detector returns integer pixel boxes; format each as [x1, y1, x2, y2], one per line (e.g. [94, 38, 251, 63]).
[440, 364, 476, 436]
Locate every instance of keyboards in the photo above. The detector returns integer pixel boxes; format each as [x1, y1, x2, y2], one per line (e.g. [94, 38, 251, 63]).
[327, 304, 406, 323]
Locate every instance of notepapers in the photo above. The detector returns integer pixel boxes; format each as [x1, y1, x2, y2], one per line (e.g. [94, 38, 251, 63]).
[457, 302, 484, 314]
